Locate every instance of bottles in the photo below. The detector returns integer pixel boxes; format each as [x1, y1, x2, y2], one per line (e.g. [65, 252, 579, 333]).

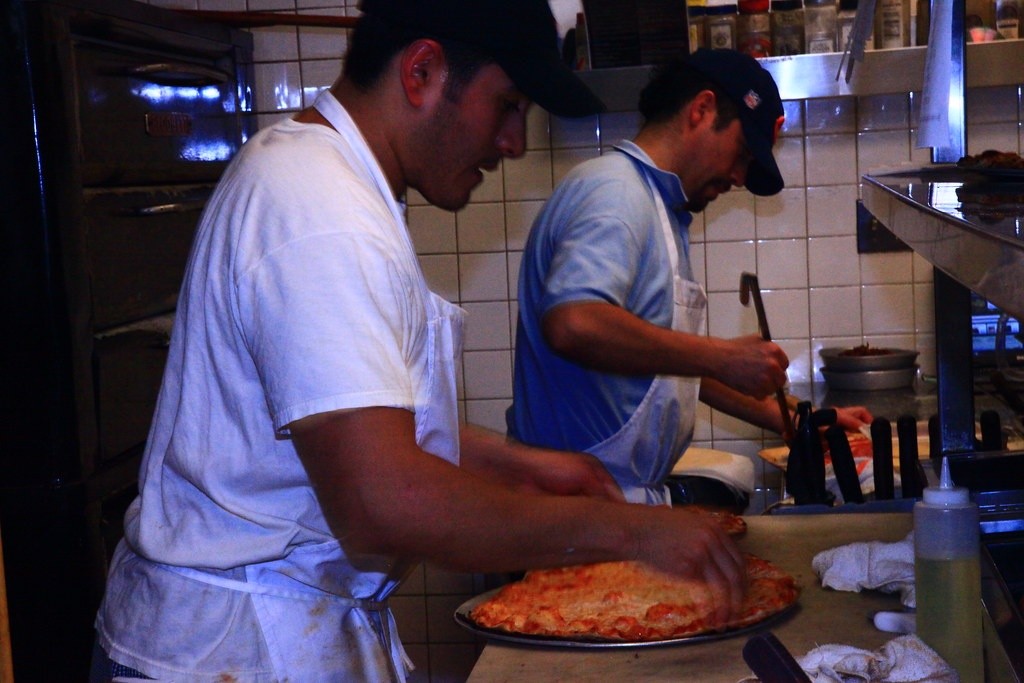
[915, 456, 984, 683]
[686, 0, 914, 59]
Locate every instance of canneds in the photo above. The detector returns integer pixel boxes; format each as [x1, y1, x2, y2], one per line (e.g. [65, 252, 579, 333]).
[686, 0, 909, 57]
[575, 12, 589, 69]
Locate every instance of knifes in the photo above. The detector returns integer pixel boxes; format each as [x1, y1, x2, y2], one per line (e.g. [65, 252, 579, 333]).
[785, 401, 1003, 506]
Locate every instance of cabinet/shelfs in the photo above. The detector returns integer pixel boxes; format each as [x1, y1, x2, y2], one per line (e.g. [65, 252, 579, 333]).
[0, 0, 259, 683]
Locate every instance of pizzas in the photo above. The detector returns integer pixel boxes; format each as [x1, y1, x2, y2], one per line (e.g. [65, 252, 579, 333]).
[468, 548, 800, 639]
[670, 506, 749, 537]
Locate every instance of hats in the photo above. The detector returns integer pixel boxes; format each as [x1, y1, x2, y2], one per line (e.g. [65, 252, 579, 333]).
[357, 0, 609, 119]
[689, 43, 785, 197]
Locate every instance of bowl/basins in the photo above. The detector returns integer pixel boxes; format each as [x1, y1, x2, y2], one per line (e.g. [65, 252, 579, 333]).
[859, 419, 982, 466]
[819, 347, 920, 370]
[820, 365, 920, 391]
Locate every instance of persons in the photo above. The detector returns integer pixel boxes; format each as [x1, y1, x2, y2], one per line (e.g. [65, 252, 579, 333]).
[507, 47, 869, 512]
[94, 0, 752, 683]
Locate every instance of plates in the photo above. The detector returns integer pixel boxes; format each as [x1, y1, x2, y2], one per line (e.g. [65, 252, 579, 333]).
[455, 588, 793, 648]
